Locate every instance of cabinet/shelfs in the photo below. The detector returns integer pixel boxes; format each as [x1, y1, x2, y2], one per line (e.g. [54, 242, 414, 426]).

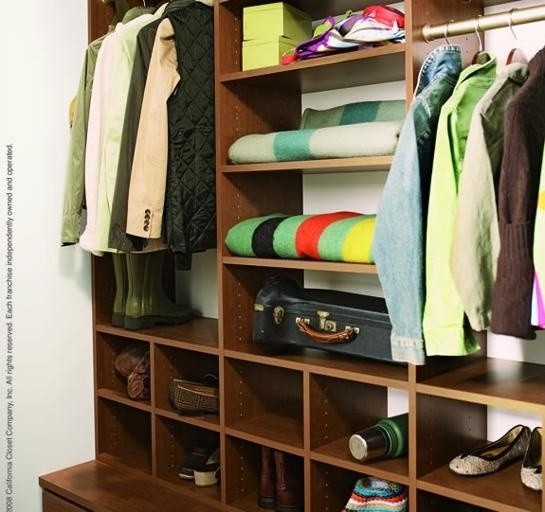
[40, 1, 543, 511]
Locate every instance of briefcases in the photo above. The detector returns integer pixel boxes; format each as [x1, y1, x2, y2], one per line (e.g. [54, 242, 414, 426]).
[251, 276, 392, 361]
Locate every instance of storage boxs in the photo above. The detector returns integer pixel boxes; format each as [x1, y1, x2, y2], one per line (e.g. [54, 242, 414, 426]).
[240, 39, 295, 68]
[236, 3, 313, 41]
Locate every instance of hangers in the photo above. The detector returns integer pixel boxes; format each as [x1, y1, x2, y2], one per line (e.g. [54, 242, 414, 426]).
[441, 20, 454, 47]
[121, 0, 157, 26]
[472, 14, 491, 63]
[102, 0, 119, 34]
[505, 7, 528, 66]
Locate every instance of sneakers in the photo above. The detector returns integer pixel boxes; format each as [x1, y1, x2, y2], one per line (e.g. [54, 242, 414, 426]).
[169, 373, 219, 416]
[449, 424, 529, 476]
[520, 427, 543, 491]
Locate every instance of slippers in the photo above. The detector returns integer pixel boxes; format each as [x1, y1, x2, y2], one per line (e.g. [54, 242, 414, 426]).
[178, 446, 220, 488]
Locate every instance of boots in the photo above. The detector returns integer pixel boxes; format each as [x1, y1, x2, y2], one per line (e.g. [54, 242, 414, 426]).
[111, 253, 195, 331]
[257, 446, 303, 511]
